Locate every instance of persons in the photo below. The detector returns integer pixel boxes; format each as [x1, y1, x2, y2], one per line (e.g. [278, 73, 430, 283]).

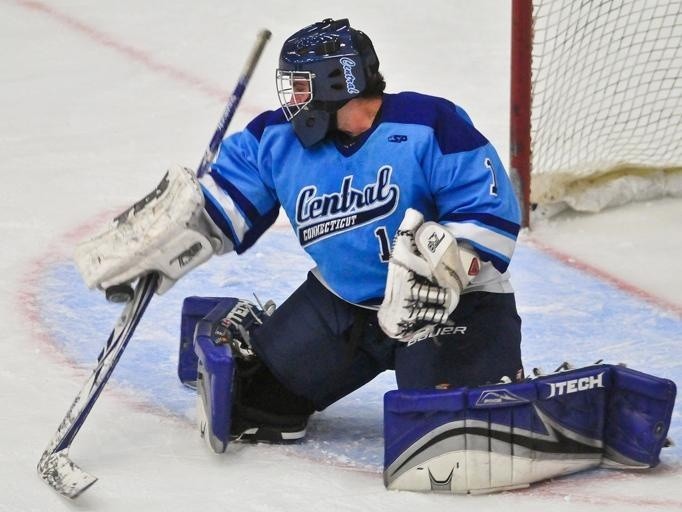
[75, 17, 676, 497]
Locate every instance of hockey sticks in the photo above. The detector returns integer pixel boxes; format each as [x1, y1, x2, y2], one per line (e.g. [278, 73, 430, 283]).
[37, 29, 270, 499]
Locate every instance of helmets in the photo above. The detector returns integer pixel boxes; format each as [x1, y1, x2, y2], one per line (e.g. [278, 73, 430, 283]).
[274, 16, 385, 149]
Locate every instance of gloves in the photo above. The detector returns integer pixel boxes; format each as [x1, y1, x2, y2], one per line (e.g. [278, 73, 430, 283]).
[70, 161, 223, 305]
[376, 205, 483, 348]
